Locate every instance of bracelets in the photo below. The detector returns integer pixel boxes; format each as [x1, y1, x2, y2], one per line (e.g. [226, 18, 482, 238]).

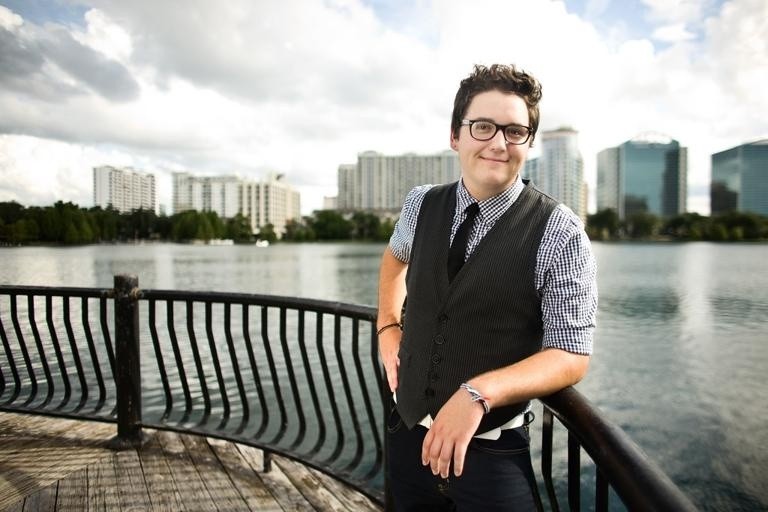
[459, 382, 489, 414]
[377, 324, 399, 336]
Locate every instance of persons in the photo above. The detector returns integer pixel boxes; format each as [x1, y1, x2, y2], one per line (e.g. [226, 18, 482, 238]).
[377, 63, 598, 511]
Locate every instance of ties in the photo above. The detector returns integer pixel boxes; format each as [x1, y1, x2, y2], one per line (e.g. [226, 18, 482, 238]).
[447, 203, 480, 280]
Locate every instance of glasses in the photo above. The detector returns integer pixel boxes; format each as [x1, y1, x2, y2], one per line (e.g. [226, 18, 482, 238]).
[461, 118, 536, 144]
